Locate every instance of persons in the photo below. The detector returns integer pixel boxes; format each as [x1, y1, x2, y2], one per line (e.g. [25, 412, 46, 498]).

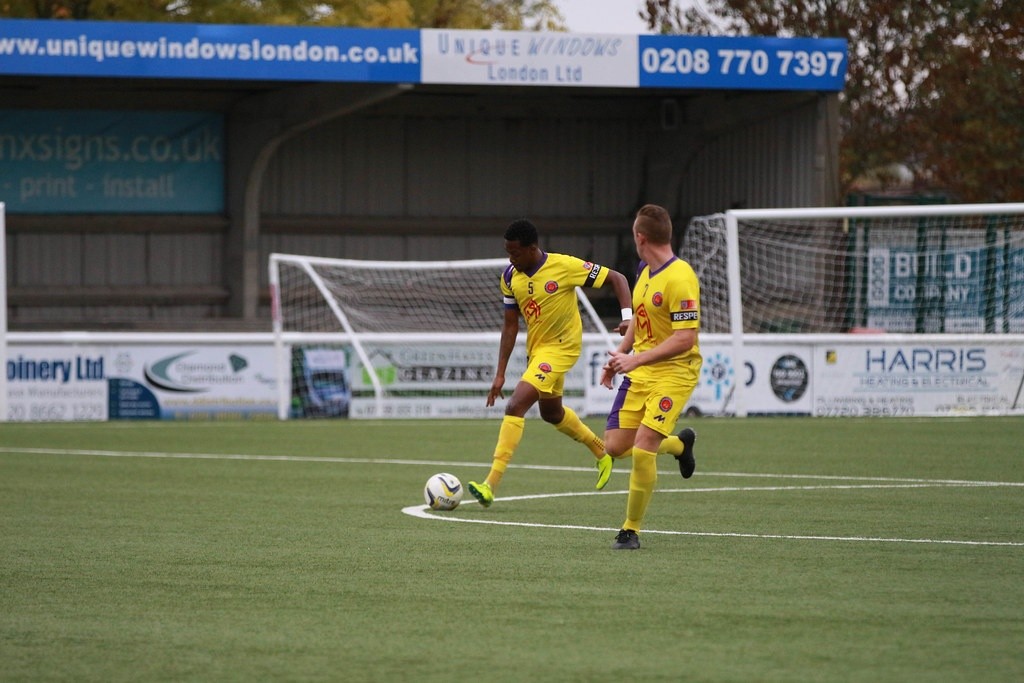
[468, 219, 633, 509]
[599, 204, 703, 549]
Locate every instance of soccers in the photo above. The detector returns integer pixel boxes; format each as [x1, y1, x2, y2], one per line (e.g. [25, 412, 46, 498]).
[424, 472, 465, 512]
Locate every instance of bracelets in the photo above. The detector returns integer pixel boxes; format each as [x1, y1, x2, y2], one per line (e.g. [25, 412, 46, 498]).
[621, 308, 633, 320]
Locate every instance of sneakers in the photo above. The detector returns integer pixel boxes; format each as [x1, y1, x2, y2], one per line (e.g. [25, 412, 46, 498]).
[468, 482, 494, 508]
[596, 453, 614, 490]
[615, 529, 641, 549]
[675, 428, 696, 479]
